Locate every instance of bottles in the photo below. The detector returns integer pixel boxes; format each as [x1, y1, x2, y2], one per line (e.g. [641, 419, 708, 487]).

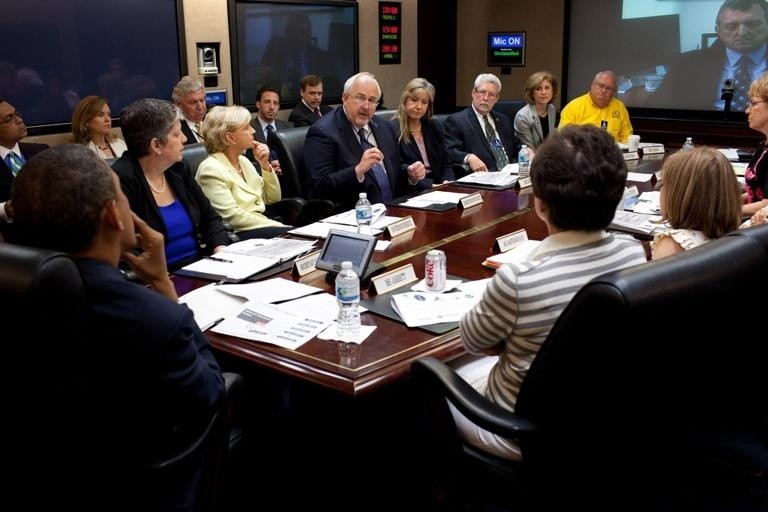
[354, 192, 374, 237]
[333, 258, 361, 338]
[516, 143, 530, 179]
[681, 137, 694, 152]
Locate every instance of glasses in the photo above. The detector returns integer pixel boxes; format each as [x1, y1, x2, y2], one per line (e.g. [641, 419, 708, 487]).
[474, 86, 498, 99]
[745, 95, 767, 109]
[347, 90, 378, 106]
[0, 111, 21, 124]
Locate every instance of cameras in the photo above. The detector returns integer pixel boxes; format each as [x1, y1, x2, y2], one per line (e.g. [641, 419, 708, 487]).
[720, 79, 737, 94]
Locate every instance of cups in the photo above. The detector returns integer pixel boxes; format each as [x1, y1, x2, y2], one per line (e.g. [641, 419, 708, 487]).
[626, 133, 641, 152]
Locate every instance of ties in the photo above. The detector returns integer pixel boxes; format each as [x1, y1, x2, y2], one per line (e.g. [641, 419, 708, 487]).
[484, 115, 508, 169]
[730, 56, 752, 111]
[264, 124, 278, 143]
[10, 150, 25, 177]
[359, 129, 391, 205]
[195, 122, 205, 143]
[314, 109, 323, 120]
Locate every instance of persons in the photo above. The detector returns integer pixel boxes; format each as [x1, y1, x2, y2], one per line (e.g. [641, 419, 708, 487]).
[0, 67, 768, 270]
[9, 140, 292, 512]
[646, 0, 768, 112]
[442, 123, 650, 466]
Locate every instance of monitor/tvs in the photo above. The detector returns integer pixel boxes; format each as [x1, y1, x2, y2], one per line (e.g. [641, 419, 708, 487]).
[315, 228, 387, 289]
[226, 1, 359, 114]
[594, 14, 682, 95]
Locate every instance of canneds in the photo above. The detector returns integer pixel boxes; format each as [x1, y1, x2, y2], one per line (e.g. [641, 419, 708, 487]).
[425, 248, 447, 293]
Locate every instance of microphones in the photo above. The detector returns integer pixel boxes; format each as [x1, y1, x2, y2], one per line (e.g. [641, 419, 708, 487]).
[367, 128, 376, 148]
